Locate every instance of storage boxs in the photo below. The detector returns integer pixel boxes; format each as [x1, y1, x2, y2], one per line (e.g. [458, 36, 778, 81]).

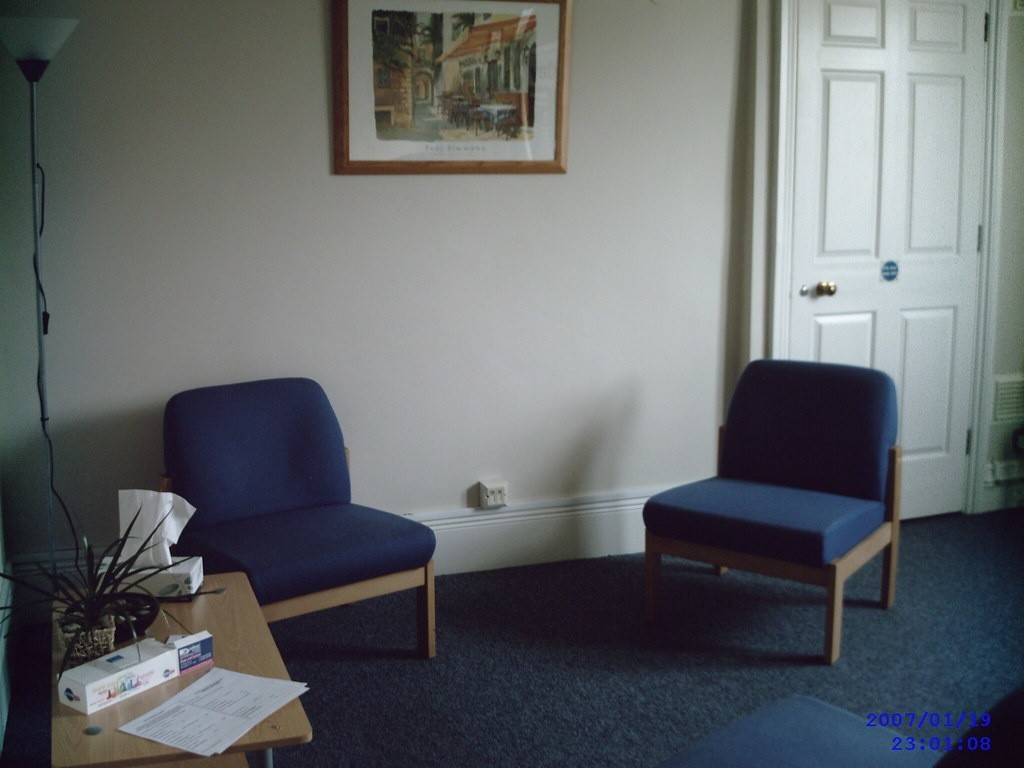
[55, 638, 181, 715]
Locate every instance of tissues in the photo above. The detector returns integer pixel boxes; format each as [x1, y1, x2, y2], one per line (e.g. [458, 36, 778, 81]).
[94, 489, 204, 603]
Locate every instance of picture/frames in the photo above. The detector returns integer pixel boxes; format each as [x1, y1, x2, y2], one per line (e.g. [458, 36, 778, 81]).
[334, 0, 573, 175]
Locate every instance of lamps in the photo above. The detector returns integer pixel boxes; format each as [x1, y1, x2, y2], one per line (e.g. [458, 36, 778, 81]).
[0, 17, 81, 576]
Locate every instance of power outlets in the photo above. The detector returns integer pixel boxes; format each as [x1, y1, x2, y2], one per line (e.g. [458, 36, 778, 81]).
[479, 479, 509, 508]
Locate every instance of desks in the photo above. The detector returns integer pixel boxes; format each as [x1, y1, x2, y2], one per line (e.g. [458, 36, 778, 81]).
[50, 571, 312, 768]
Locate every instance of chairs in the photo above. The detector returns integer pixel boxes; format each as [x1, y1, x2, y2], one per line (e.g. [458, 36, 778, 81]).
[640, 360, 902, 668]
[161, 375, 437, 658]
